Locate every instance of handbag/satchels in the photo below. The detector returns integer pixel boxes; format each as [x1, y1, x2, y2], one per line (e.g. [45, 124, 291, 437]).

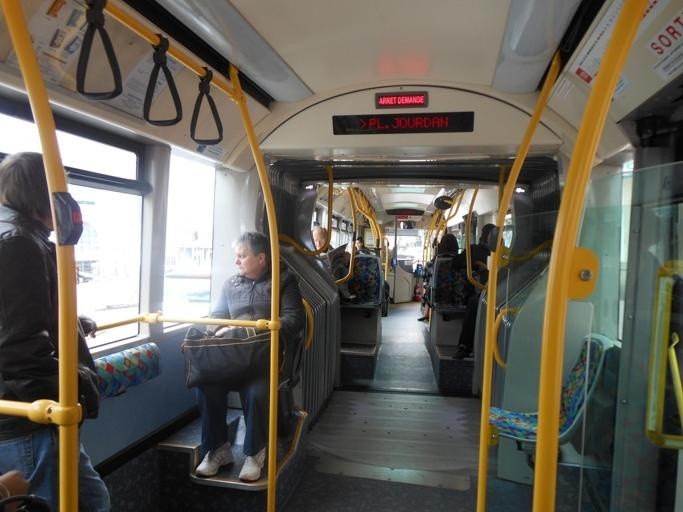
[181, 326, 283, 389]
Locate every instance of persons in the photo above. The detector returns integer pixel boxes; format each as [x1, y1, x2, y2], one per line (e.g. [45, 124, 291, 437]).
[195, 231, 305, 481]
[417, 224, 510, 359]
[0, 152, 111, 512]
[311, 225, 369, 262]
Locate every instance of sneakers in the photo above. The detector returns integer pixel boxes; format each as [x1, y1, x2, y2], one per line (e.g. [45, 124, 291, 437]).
[238, 447, 266, 481]
[196, 442, 234, 476]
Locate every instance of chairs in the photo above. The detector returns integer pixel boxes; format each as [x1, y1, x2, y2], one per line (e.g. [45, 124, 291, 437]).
[488, 333, 613, 487]
[343, 249, 381, 345]
[422, 255, 466, 347]
[266, 298, 314, 422]
